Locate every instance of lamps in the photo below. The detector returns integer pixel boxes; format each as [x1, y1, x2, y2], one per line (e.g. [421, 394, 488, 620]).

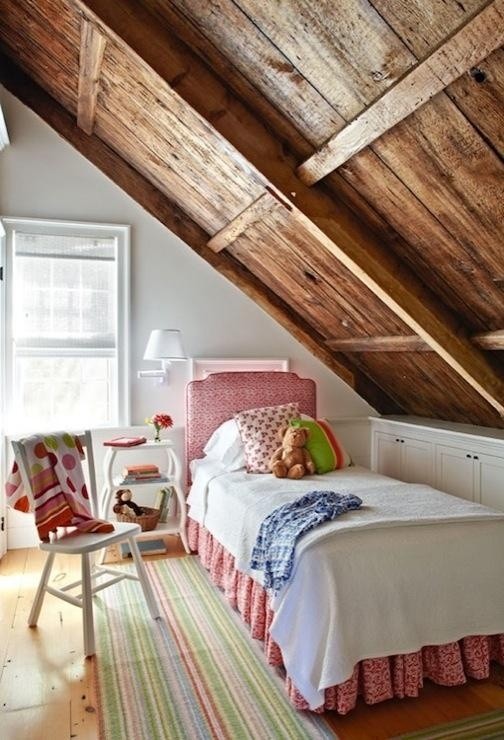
[136, 328, 188, 385]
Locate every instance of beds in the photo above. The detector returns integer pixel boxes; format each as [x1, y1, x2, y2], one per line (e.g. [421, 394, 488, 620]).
[182, 371, 504, 715]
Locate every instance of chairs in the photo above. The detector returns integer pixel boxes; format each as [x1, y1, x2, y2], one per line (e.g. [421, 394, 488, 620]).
[9, 430, 161, 658]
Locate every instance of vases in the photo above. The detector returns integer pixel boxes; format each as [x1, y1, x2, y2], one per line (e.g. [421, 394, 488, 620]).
[153, 432, 160, 443]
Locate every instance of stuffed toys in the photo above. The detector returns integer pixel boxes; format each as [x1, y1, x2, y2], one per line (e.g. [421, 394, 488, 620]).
[270, 426, 318, 477]
[111, 489, 155, 519]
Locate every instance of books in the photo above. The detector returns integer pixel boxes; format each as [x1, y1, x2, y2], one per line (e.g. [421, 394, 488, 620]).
[119, 540, 168, 558]
[119, 465, 171, 485]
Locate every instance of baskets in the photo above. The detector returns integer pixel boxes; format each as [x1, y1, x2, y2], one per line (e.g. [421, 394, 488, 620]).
[117, 507, 162, 531]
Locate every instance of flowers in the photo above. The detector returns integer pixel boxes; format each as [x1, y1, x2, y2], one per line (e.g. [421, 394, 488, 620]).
[144, 411, 176, 441]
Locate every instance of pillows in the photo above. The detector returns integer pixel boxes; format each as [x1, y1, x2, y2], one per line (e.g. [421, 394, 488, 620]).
[201, 408, 314, 474]
[290, 416, 352, 475]
[231, 401, 299, 474]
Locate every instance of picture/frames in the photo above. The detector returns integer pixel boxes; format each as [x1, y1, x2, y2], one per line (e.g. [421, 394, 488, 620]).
[101, 435, 146, 447]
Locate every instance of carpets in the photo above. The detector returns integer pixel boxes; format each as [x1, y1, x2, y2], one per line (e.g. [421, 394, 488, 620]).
[83, 554, 340, 739]
[374, 708, 502, 739]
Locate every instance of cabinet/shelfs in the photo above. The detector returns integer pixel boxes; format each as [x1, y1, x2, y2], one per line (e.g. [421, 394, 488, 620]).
[435, 430, 503, 511]
[367, 417, 434, 485]
[97, 437, 190, 564]
